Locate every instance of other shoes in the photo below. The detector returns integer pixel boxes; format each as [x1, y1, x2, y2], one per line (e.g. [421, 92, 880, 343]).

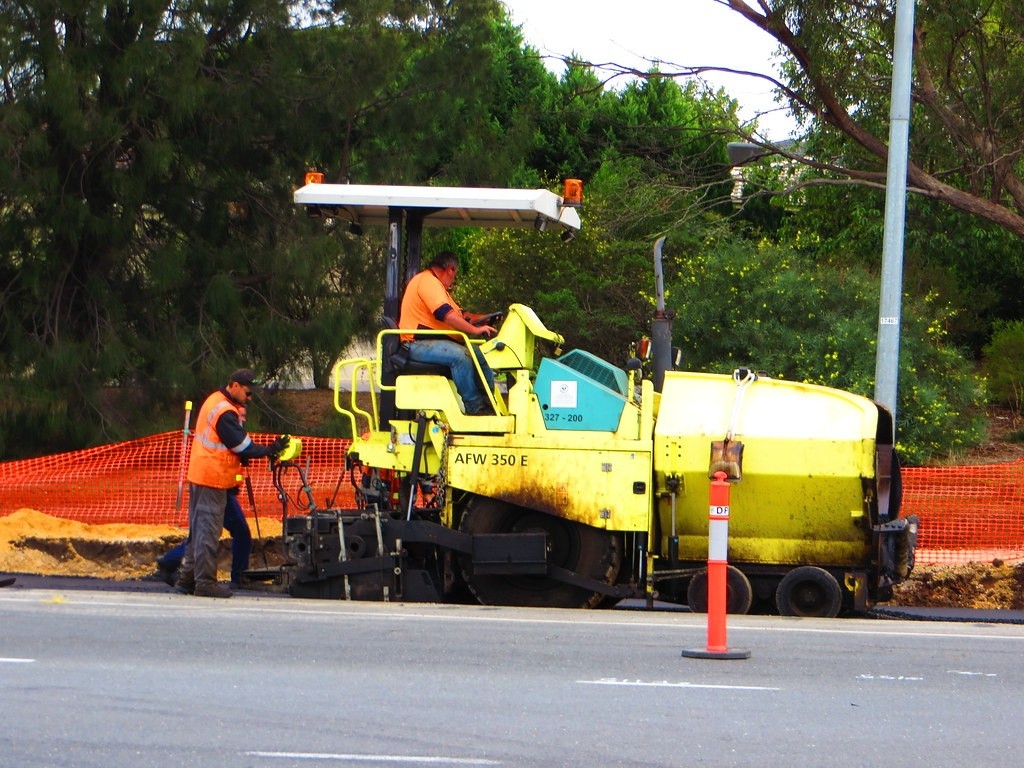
[194, 583, 233, 598]
[175, 579, 195, 594]
[156, 556, 180, 588]
[229, 582, 245, 589]
[467, 404, 496, 416]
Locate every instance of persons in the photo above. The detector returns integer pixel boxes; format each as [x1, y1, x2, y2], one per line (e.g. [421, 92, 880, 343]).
[399, 250, 504, 416]
[156, 368, 290, 598]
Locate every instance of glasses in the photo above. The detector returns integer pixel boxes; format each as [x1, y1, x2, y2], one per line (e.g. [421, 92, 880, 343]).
[241, 386, 256, 397]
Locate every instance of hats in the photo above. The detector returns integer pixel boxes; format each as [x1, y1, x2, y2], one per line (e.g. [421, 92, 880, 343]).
[231, 369, 261, 388]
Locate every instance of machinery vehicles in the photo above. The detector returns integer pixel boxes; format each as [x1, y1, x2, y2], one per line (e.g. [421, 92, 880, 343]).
[236, 170, 923, 620]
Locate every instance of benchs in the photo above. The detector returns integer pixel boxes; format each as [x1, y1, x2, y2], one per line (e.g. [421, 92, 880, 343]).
[379, 315, 452, 378]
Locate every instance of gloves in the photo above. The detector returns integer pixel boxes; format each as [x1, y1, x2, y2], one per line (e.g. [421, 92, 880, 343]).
[267, 435, 290, 456]
